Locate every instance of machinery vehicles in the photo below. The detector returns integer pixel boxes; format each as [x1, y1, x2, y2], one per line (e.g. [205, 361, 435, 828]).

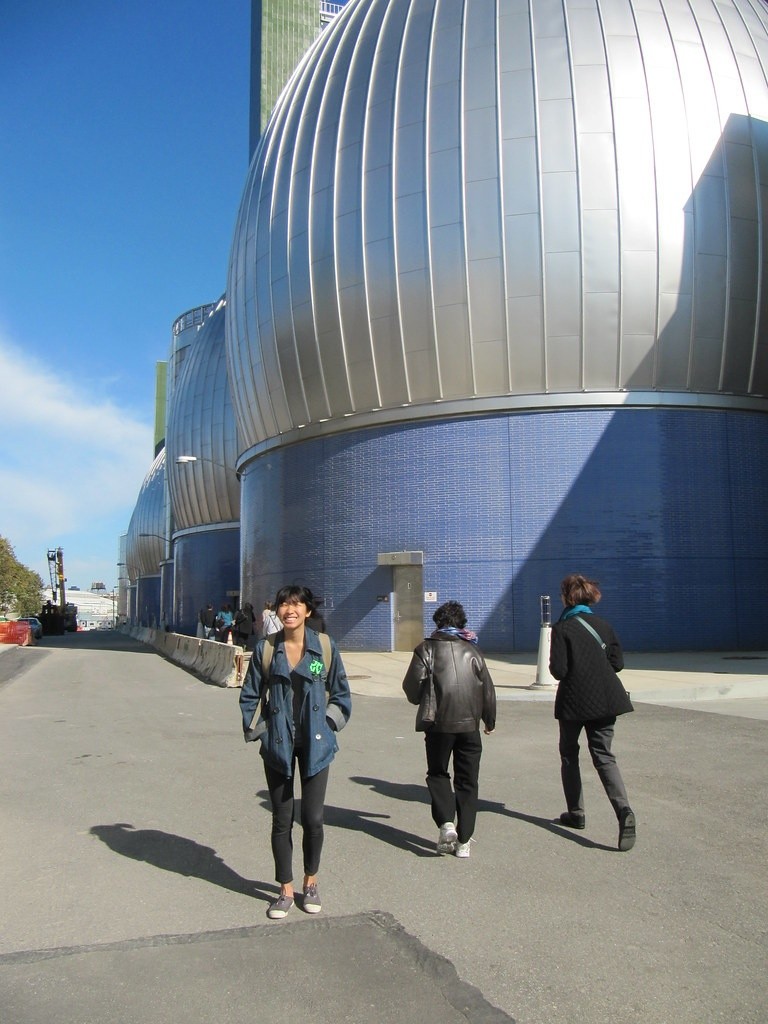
[47, 547, 78, 633]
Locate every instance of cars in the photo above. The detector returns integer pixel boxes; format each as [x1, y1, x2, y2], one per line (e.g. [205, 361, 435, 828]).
[18, 618, 43, 638]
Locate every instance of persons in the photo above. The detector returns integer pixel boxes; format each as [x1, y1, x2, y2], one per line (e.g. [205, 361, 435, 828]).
[236, 603, 255, 650]
[240, 585, 351, 920]
[549, 574, 638, 852]
[201, 603, 219, 640]
[402, 599, 496, 859]
[263, 599, 274, 622]
[217, 604, 234, 641]
[264, 603, 284, 636]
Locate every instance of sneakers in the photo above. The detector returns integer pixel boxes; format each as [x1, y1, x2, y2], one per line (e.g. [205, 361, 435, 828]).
[435, 823, 470, 858]
[267, 885, 321, 919]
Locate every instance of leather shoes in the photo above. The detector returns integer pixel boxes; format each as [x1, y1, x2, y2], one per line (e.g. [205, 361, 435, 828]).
[561, 812, 585, 828]
[618, 807, 636, 851]
